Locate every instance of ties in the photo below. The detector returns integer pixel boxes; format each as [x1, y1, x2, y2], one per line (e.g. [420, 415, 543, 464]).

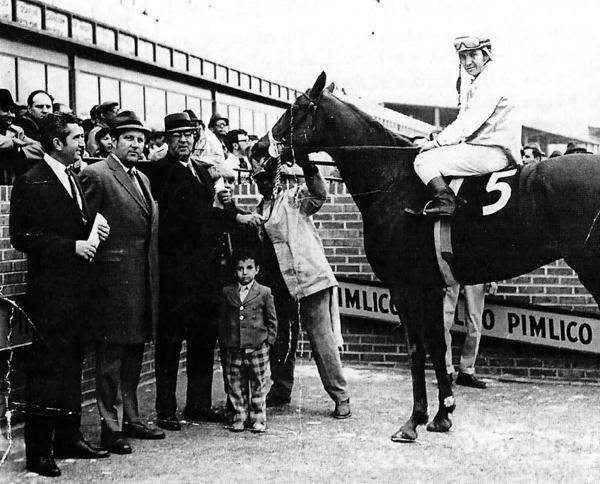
[128, 171, 144, 203]
[65, 169, 79, 210]
[186, 163, 200, 182]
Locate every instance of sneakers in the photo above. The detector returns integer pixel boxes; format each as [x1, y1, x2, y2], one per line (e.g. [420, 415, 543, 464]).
[266, 391, 291, 407]
[230, 418, 268, 433]
[447, 372, 488, 390]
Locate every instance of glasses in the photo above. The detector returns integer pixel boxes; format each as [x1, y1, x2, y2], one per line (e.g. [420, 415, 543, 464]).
[238, 139, 251, 143]
[453, 39, 490, 50]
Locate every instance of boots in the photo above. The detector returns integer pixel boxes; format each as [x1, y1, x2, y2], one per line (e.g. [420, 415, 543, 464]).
[403, 176, 457, 221]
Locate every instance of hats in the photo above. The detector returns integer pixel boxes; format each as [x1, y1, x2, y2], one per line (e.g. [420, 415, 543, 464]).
[208, 164, 236, 180]
[107, 109, 152, 136]
[0, 88, 229, 129]
[160, 112, 202, 137]
[520, 142, 594, 158]
[452, 34, 491, 52]
[251, 166, 267, 179]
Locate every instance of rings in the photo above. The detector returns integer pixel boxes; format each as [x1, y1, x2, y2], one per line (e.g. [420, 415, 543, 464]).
[85, 250, 89, 253]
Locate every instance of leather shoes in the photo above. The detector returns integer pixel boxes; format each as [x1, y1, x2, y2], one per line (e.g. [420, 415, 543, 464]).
[334, 396, 351, 419]
[52, 440, 111, 459]
[101, 431, 132, 454]
[156, 412, 182, 431]
[184, 405, 223, 423]
[25, 454, 61, 478]
[122, 422, 166, 439]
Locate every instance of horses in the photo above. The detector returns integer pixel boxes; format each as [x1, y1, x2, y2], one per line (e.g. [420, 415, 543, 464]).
[251, 67, 599, 444]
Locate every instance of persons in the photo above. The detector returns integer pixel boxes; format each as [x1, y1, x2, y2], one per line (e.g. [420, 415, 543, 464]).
[523, 146, 542, 165]
[0, 89, 259, 208]
[77, 111, 165, 454]
[8, 111, 110, 476]
[219, 152, 352, 419]
[405, 33, 522, 217]
[218, 248, 279, 433]
[443, 281, 500, 388]
[144, 112, 268, 430]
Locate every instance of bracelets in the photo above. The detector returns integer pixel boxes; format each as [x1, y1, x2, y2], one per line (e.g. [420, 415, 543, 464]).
[433, 139, 441, 147]
